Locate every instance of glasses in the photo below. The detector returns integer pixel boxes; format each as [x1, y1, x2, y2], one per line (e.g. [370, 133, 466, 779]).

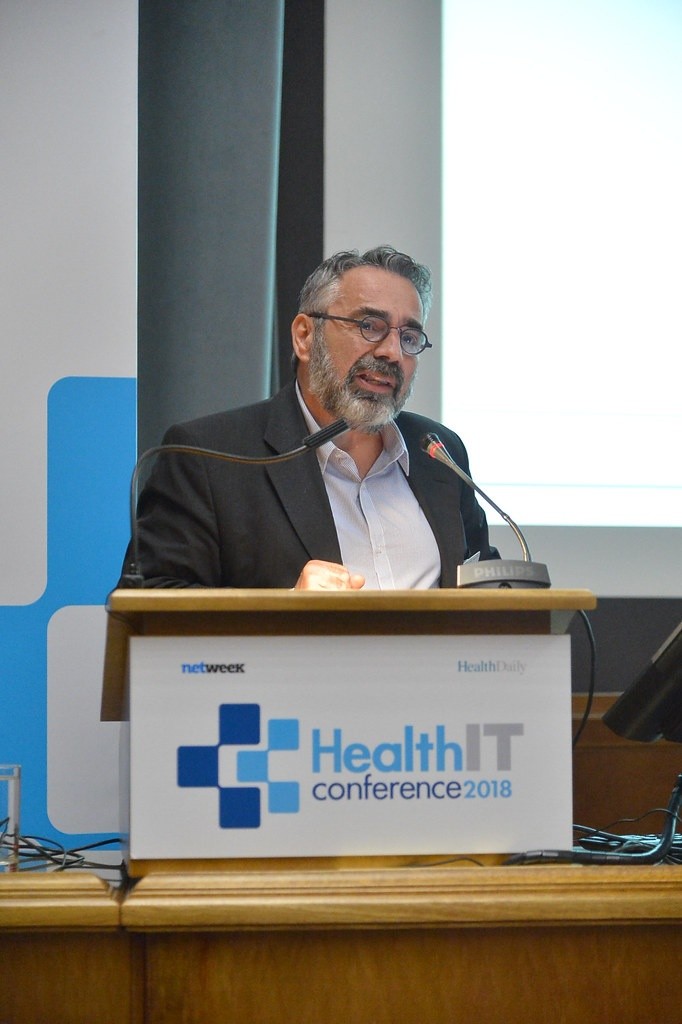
[308, 312, 433, 356]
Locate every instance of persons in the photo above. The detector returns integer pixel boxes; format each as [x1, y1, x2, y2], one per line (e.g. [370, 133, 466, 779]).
[118, 245, 505, 591]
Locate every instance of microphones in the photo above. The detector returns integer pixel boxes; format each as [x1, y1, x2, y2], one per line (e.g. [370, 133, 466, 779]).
[119, 419, 349, 589]
[418, 432, 552, 590]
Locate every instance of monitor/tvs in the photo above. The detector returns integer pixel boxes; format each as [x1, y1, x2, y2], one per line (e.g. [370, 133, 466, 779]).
[600, 621, 682, 745]
